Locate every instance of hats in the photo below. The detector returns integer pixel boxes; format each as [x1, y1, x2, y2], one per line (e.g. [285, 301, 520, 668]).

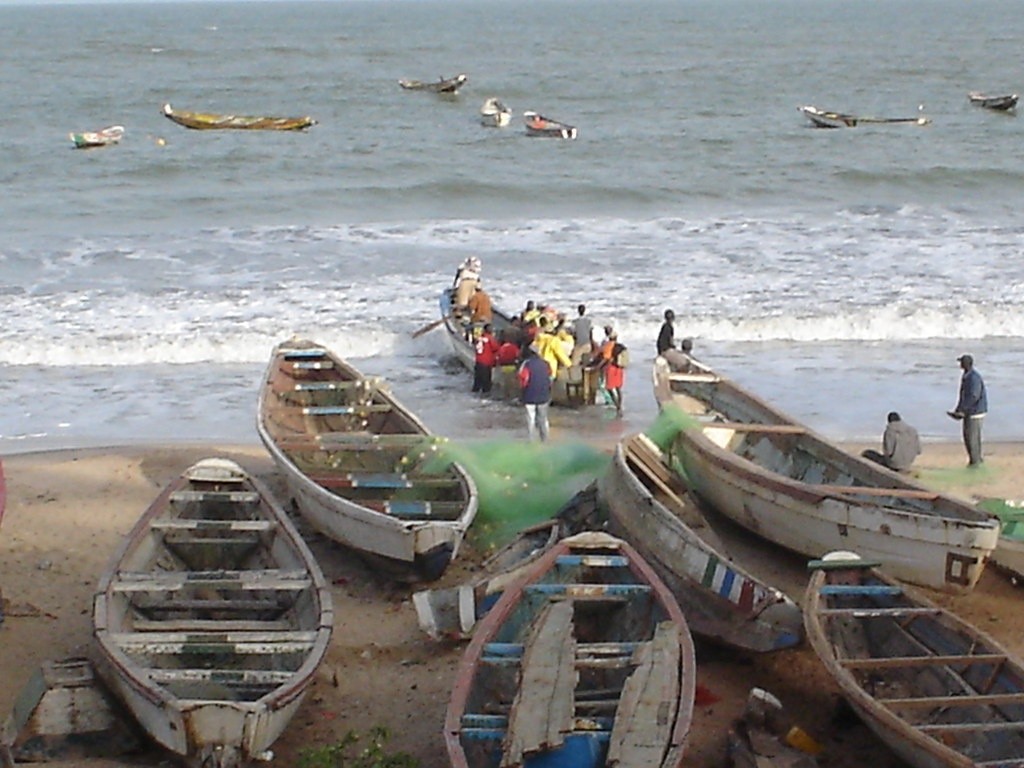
[957, 356, 972, 364]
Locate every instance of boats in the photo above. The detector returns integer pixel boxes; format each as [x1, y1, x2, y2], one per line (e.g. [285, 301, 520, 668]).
[398, 73, 468, 94]
[444, 531, 696, 768]
[948, 499, 1024, 586]
[967, 90, 1018, 111]
[798, 105, 933, 127]
[602, 431, 805, 656]
[724, 688, 827, 768]
[256, 332, 477, 583]
[163, 103, 318, 131]
[412, 479, 600, 642]
[68, 126, 125, 148]
[802, 550, 1024, 768]
[0, 457, 332, 768]
[652, 350, 1001, 595]
[523, 111, 577, 139]
[482, 98, 513, 128]
[441, 288, 600, 409]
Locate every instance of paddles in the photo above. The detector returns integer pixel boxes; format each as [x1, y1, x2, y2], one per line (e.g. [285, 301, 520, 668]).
[412, 309, 464, 340]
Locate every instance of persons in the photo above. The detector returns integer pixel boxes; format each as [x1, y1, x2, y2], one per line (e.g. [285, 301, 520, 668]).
[954, 355, 988, 469]
[497, 301, 575, 380]
[877, 412, 922, 472]
[572, 305, 594, 360]
[596, 325, 624, 411]
[657, 310, 676, 355]
[515, 344, 552, 441]
[471, 324, 499, 397]
[469, 284, 492, 324]
[456, 272, 477, 303]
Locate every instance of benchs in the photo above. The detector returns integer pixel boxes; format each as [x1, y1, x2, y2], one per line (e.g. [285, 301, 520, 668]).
[690, 420, 806, 433]
[813, 484, 939, 501]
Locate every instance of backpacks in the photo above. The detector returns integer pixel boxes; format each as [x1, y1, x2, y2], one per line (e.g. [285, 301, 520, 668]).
[610, 343, 628, 367]
